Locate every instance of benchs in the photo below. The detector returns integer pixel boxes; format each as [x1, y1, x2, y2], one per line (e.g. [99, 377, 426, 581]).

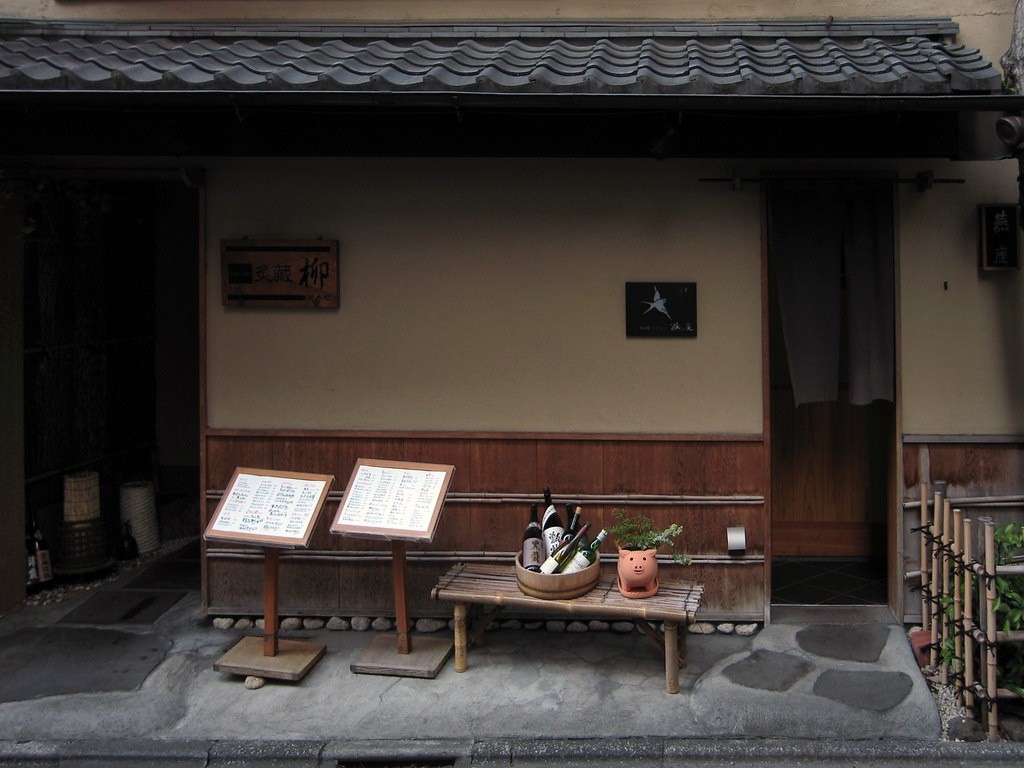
[432, 562, 706, 696]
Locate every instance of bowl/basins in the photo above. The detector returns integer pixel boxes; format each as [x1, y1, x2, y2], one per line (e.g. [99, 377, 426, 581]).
[514, 549, 601, 599]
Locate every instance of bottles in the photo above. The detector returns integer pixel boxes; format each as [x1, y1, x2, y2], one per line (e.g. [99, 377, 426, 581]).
[539, 521, 592, 575]
[521, 502, 546, 573]
[541, 486, 565, 561]
[30, 517, 53, 583]
[560, 507, 582, 547]
[558, 529, 610, 574]
[565, 503, 589, 550]
